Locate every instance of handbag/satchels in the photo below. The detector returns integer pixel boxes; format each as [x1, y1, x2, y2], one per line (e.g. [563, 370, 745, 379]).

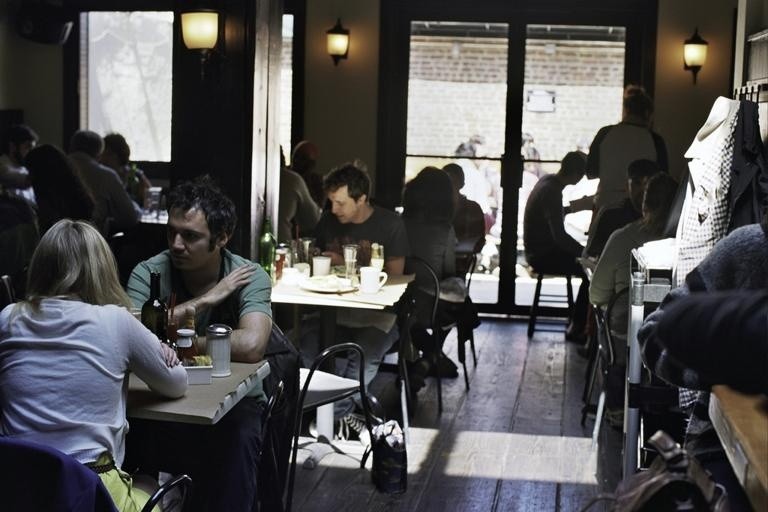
[371, 421, 408, 492]
[582, 431, 732, 512]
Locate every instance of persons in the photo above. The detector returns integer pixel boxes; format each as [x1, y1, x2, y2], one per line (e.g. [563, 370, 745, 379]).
[1, 112, 487, 512]
[449, 134, 545, 277]
[523, 152, 592, 346]
[563, 85, 683, 427]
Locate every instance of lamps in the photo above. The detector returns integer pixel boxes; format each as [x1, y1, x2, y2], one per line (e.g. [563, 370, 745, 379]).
[180, 0, 221, 66]
[683, 24, 708, 85]
[325, 16, 350, 67]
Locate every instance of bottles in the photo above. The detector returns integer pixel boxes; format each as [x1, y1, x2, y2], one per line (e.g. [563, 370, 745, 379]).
[257, 215, 275, 288]
[139, 272, 167, 345]
[180, 305, 196, 328]
[174, 328, 198, 359]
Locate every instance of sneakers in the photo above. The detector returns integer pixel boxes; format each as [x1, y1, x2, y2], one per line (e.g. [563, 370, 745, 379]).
[309, 414, 371, 441]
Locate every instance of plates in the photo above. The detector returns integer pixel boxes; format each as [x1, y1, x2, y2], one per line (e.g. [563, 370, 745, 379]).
[302, 282, 357, 296]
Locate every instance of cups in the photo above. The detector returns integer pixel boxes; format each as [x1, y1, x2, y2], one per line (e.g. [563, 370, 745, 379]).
[311, 255, 331, 277]
[341, 243, 357, 277]
[163, 314, 179, 343]
[357, 266, 388, 295]
[205, 324, 233, 378]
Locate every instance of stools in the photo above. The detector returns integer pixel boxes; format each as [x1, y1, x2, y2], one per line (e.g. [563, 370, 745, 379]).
[527, 267, 577, 339]
